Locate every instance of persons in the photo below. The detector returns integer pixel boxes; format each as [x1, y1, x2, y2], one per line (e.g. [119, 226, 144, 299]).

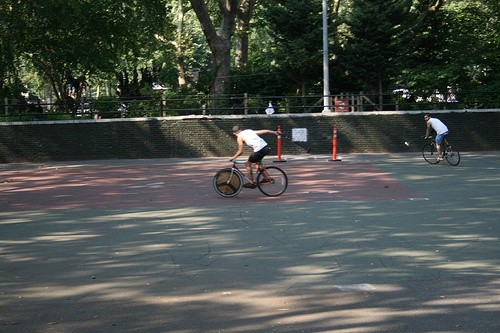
[93, 113, 102, 119]
[229, 125, 282, 189]
[424, 113, 452, 160]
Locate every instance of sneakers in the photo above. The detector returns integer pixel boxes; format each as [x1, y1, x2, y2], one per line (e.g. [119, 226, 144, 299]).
[436, 157, 443, 160]
[259, 178, 274, 184]
[241, 182, 256, 189]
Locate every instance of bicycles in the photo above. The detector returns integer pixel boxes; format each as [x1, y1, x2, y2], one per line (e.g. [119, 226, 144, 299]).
[213, 157, 288, 197]
[422, 136, 460, 166]
